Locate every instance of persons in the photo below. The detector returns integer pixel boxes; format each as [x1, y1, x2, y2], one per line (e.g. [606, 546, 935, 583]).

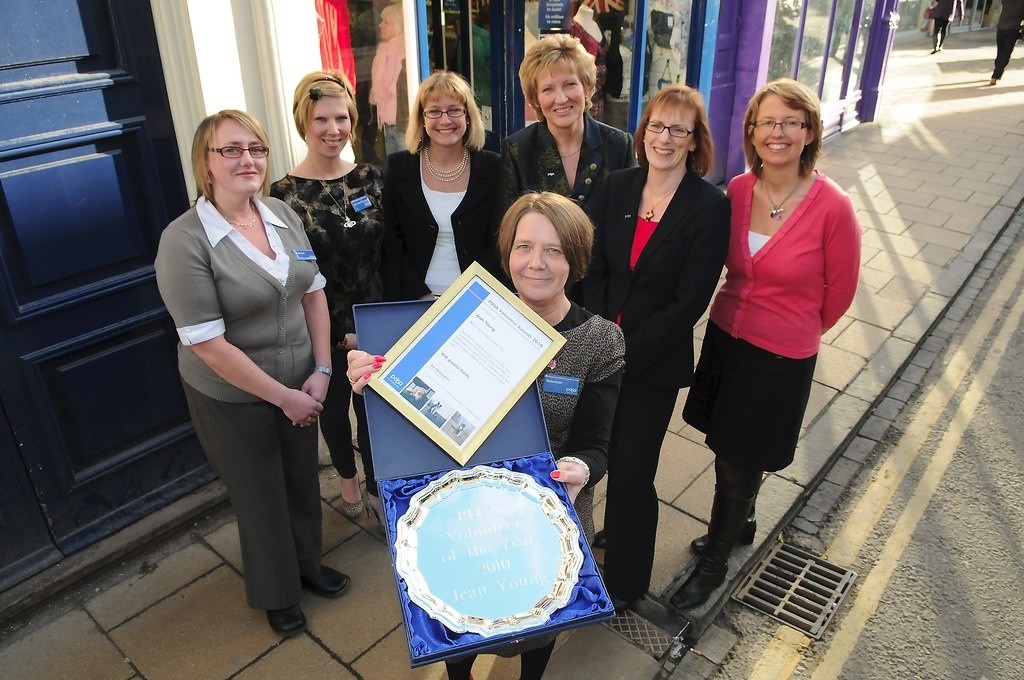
[930, 0, 964, 55]
[666, 76, 863, 609]
[572, 4, 607, 124]
[366, 2, 413, 155]
[345, 190, 625, 680]
[498, 33, 636, 314]
[151, 111, 353, 639]
[379, 69, 507, 300]
[603, 13, 651, 134]
[649, 4, 682, 100]
[584, 85, 733, 611]
[269, 70, 390, 541]
[456, 5, 494, 107]
[989, 0, 1024, 84]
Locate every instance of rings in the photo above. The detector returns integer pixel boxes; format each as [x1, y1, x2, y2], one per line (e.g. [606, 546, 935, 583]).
[348, 377, 355, 384]
[306, 416, 312, 422]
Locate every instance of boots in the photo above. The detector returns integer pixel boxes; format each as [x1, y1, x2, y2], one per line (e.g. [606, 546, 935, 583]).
[692, 495, 757, 554]
[670, 490, 755, 612]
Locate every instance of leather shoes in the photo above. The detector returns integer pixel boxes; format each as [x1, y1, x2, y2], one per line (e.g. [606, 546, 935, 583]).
[269, 605, 306, 634]
[300, 566, 350, 597]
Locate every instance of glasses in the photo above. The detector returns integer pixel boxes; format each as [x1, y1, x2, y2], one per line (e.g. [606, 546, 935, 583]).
[423, 108, 467, 119]
[644, 120, 697, 138]
[207, 144, 269, 158]
[753, 120, 808, 131]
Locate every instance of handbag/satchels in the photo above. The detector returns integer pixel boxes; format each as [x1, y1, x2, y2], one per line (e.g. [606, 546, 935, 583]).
[925, 6, 935, 18]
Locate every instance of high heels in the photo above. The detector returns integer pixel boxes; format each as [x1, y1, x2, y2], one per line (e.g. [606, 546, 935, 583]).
[364, 491, 386, 533]
[341, 472, 363, 519]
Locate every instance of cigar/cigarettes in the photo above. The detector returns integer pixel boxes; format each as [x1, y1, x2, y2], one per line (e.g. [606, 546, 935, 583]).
[690, 648, 701, 655]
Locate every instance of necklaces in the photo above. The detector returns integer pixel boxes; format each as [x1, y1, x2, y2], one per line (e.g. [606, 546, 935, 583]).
[314, 167, 357, 228]
[562, 148, 580, 157]
[224, 201, 257, 229]
[424, 146, 469, 182]
[644, 186, 675, 221]
[761, 172, 801, 221]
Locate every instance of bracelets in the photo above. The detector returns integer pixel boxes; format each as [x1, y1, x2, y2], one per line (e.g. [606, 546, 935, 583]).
[315, 366, 332, 376]
[555, 457, 590, 489]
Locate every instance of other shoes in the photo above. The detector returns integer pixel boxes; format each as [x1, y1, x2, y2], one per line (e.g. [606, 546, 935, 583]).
[594, 529, 606, 548]
[939, 45, 942, 51]
[609, 592, 631, 611]
[931, 49, 938, 54]
[991, 77, 996, 85]
